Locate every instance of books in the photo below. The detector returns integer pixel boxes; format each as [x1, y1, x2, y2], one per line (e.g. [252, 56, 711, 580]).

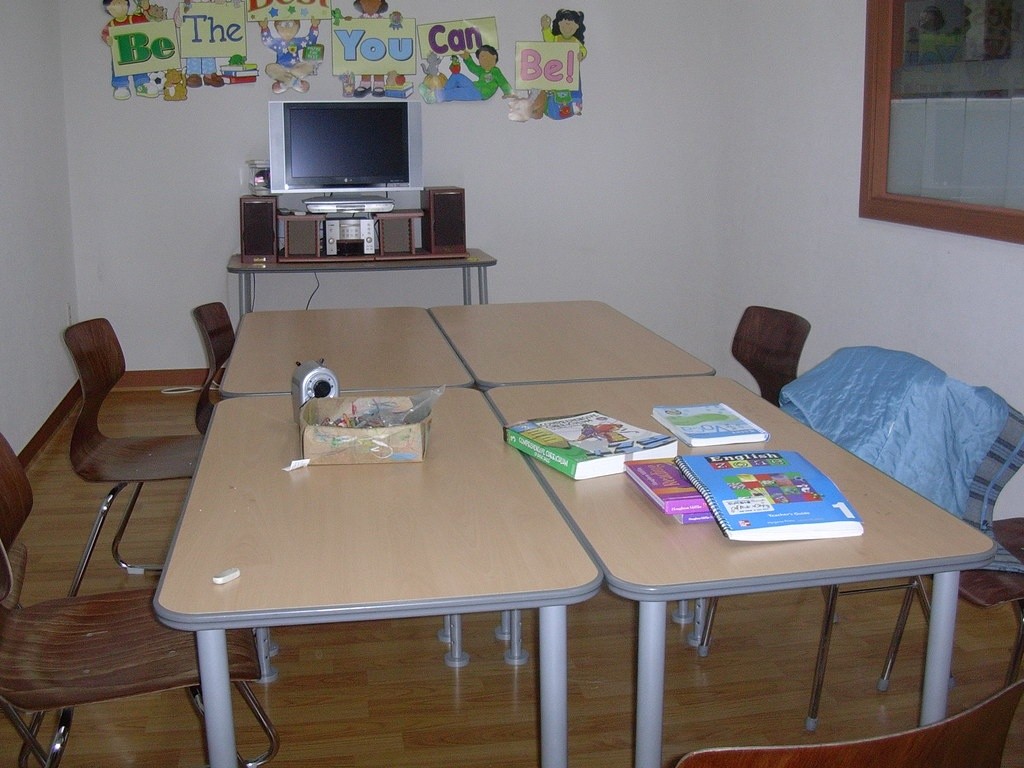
[674, 450, 865, 541]
[673, 510, 715, 525]
[650, 402, 771, 448]
[502, 410, 679, 482]
[623, 456, 710, 515]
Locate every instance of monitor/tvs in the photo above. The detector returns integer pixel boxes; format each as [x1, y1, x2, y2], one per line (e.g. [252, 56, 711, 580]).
[269, 98, 423, 193]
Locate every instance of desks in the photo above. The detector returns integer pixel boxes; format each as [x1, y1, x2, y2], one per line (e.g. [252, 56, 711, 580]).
[226, 248, 497, 319]
[217, 307, 476, 657]
[484, 375, 998, 768]
[150, 386, 603, 768]
[427, 300, 718, 641]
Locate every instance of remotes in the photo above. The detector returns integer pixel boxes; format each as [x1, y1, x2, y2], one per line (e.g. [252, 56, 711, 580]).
[290, 209, 307, 216]
[278, 208, 291, 216]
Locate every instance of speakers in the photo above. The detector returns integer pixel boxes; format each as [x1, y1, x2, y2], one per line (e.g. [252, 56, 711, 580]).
[422, 186, 467, 254]
[239, 195, 277, 265]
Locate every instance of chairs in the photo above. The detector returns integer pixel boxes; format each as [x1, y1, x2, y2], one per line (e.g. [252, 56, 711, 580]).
[62, 318, 206, 598]
[0, 433, 280, 768]
[192, 302, 235, 434]
[674, 305, 1024, 768]
